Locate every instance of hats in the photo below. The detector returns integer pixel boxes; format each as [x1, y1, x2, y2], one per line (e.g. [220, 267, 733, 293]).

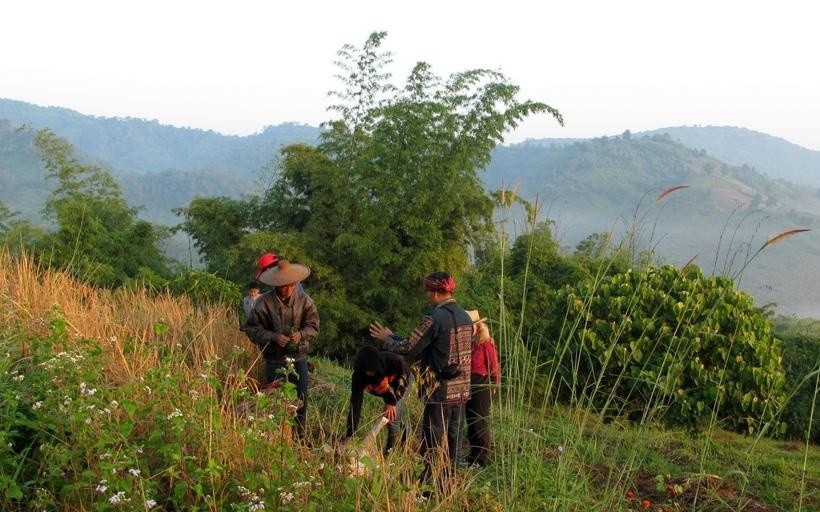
[256, 253, 279, 279]
[259, 260, 312, 287]
[464, 309, 488, 324]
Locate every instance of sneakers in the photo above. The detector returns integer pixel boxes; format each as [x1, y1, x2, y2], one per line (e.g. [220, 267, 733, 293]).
[470, 463, 483, 470]
[460, 456, 473, 466]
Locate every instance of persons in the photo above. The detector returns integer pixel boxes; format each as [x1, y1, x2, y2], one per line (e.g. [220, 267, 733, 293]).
[340, 344, 415, 459]
[231, 252, 321, 449]
[369, 271, 473, 501]
[459, 309, 502, 469]
